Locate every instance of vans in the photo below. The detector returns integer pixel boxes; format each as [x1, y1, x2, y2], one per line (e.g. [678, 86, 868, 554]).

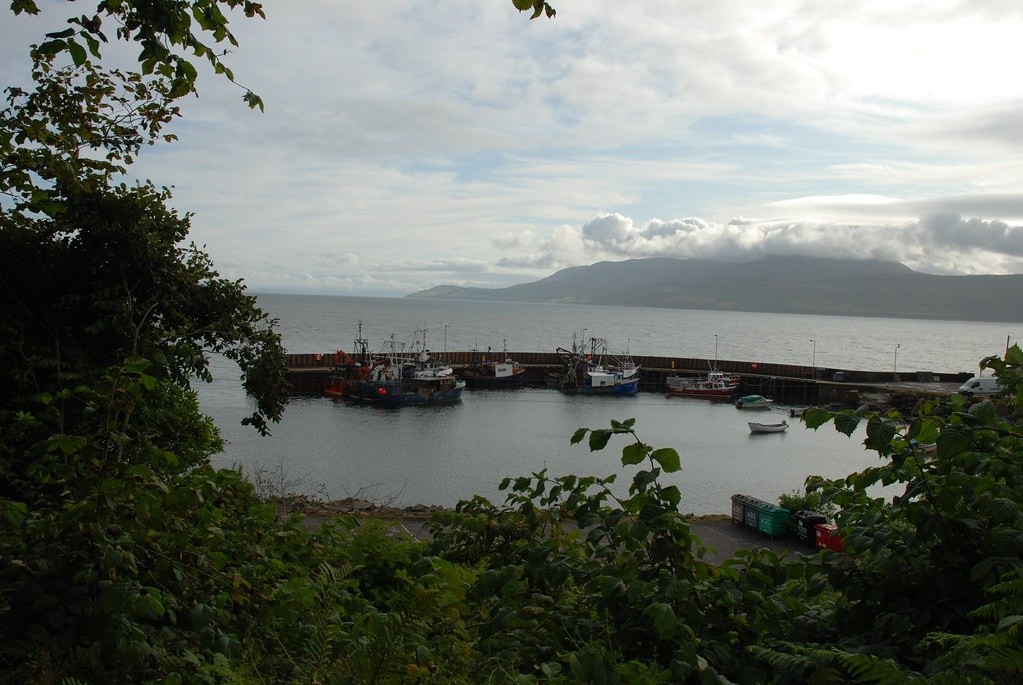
[958, 378, 1008, 398]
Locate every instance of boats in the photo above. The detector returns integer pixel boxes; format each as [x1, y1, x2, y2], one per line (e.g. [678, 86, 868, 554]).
[736, 395, 773, 409]
[666, 360, 739, 398]
[324, 319, 641, 404]
[791, 407, 818, 417]
[748, 420, 789, 433]
[903, 438, 937, 455]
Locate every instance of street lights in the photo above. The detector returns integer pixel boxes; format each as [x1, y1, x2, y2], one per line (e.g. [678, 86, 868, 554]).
[445, 325, 450, 364]
[894, 344, 900, 382]
[810, 340, 815, 380]
[715, 334, 718, 372]
[583, 328, 587, 359]
